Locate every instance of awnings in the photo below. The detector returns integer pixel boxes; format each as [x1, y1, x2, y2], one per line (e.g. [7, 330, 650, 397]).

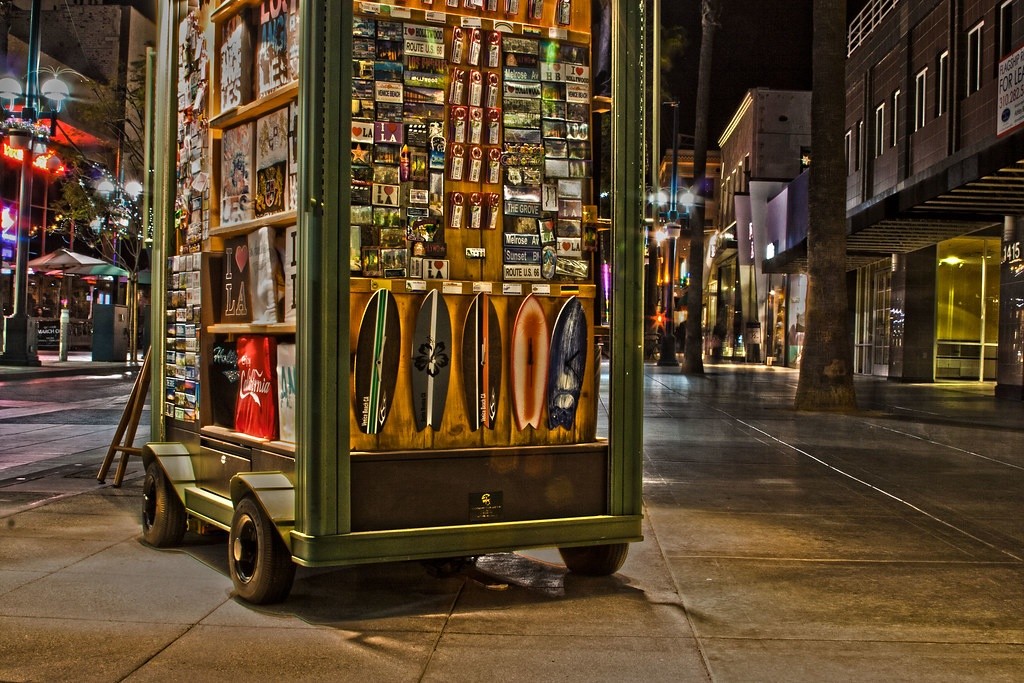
[2, 109, 109, 162]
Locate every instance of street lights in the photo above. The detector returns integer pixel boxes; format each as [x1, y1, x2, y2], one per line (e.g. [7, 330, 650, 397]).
[98, 160, 144, 305]
[0, 0, 69, 370]
[648, 98, 694, 368]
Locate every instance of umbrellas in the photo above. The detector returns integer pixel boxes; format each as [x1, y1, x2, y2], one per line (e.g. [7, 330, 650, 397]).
[8, 244, 128, 348]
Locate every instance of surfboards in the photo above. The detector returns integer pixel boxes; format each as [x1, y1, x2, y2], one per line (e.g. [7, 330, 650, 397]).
[460, 291, 502, 433]
[546, 294, 588, 431]
[353, 287, 401, 435]
[508, 292, 550, 431]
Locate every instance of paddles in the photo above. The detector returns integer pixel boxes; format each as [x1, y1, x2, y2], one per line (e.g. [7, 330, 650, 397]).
[410, 288, 452, 432]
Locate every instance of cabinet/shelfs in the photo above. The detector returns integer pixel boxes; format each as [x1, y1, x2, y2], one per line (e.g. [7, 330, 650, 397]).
[936, 339, 981, 380]
[201, 0, 298, 456]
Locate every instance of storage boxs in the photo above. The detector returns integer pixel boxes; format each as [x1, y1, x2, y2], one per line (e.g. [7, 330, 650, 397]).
[220, 0, 298, 226]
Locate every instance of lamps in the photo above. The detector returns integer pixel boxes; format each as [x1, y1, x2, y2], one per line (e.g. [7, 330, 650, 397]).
[891, 253, 899, 272]
[1004, 215, 1015, 242]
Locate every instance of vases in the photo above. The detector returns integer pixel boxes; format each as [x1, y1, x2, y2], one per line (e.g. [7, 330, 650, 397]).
[9, 128, 32, 149]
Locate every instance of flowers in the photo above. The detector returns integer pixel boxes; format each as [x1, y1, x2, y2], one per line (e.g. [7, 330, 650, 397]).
[0, 115, 50, 141]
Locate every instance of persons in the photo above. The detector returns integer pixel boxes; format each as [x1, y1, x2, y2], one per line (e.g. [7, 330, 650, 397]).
[26, 292, 38, 316]
[45, 293, 52, 303]
[43, 293, 46, 303]
